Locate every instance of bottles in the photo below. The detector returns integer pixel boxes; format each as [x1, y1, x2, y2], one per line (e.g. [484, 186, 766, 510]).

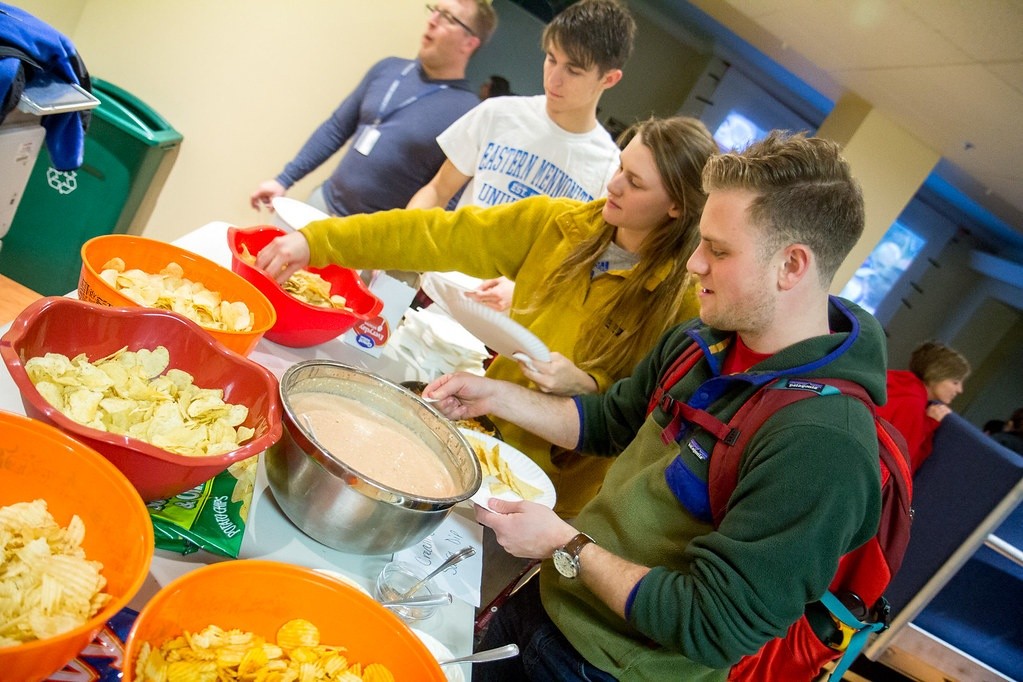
[805, 590, 868, 651]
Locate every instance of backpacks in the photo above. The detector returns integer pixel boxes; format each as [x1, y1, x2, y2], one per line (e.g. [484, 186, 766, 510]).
[648, 338, 917, 682]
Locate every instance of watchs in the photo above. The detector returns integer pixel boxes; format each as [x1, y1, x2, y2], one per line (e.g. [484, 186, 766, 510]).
[552, 531, 596, 578]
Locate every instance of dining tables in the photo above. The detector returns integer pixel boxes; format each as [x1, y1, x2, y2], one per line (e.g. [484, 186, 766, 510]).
[0, 219, 493, 682]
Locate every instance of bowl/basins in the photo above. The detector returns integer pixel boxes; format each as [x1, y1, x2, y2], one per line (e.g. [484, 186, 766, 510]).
[374, 560, 442, 624]
[271, 196, 332, 234]
[227, 224, 385, 348]
[264, 359, 482, 556]
[1, 407, 156, 682]
[0, 296, 285, 503]
[76, 234, 276, 358]
[119, 557, 449, 682]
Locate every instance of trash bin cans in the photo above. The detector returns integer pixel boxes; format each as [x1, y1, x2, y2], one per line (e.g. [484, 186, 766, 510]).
[0, 73, 184, 295]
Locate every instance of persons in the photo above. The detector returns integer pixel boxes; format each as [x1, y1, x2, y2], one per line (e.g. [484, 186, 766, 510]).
[420, 129, 887, 682]
[250, 0, 499, 218]
[405, 0, 637, 318]
[254, 116, 722, 616]
[875, 341, 971, 478]
[445, 75, 510, 211]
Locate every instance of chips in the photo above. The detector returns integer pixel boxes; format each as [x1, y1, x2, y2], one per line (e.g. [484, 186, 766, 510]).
[132, 617, 398, 682]
[463, 434, 537, 501]
[22, 345, 256, 456]
[227, 453, 258, 526]
[238, 243, 354, 312]
[97, 256, 255, 334]
[1, 498, 111, 649]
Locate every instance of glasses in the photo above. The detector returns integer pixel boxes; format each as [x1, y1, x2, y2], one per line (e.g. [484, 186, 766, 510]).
[426, 2, 479, 37]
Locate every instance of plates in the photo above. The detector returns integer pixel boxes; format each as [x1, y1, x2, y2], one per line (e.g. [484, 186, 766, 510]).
[455, 428, 556, 516]
[451, 298, 550, 363]
